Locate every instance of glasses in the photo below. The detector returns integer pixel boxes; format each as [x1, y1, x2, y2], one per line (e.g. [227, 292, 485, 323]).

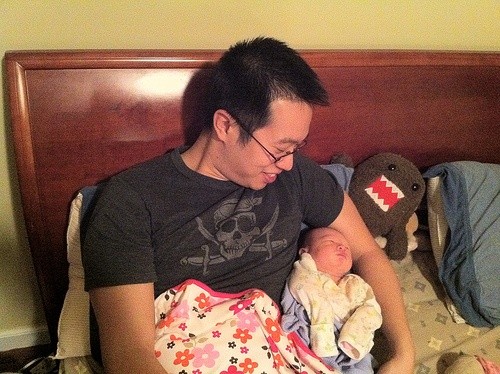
[230, 112, 308, 164]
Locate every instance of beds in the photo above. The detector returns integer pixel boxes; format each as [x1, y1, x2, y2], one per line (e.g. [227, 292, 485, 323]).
[0, 50, 500, 374]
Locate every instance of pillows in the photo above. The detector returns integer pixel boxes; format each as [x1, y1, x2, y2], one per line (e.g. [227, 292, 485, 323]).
[47, 165, 354, 359]
[422, 161, 500, 329]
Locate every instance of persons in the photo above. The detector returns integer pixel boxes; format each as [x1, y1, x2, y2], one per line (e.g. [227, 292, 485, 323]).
[83, 37, 416, 374]
[290, 226, 382, 374]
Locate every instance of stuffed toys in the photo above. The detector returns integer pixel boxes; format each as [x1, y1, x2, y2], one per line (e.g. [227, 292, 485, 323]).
[330, 152, 425, 262]
